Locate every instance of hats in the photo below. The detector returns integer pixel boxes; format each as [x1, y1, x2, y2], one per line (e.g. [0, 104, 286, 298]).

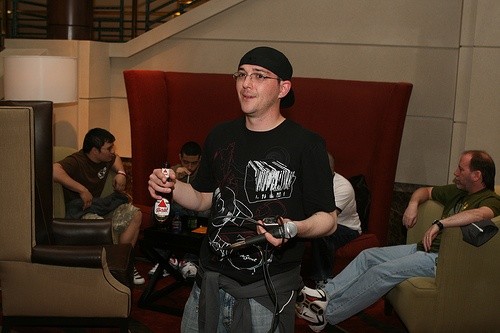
[238, 47, 295, 108]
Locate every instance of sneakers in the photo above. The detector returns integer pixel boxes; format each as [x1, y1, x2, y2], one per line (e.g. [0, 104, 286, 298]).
[149, 259, 178, 276]
[178, 260, 197, 277]
[132, 266, 145, 284]
[295, 285, 329, 332]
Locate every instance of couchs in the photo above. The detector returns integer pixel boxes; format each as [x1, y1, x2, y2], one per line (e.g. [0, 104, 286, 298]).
[125, 68, 415, 316]
[0, 100, 151, 333]
[387, 185, 500, 333]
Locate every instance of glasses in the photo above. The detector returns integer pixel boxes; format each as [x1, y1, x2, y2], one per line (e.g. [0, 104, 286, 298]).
[233, 72, 280, 83]
[101, 145, 114, 152]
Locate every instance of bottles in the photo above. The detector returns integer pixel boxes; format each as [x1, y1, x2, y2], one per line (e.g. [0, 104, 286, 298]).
[152, 160, 173, 229]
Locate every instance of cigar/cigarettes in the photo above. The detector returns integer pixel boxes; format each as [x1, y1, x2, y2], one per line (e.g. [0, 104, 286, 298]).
[188, 175, 190, 184]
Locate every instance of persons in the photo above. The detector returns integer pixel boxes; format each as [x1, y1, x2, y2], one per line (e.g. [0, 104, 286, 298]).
[296, 150, 500, 333]
[149, 142, 203, 278]
[148, 46, 337, 333]
[52, 128, 146, 285]
[300, 152, 362, 288]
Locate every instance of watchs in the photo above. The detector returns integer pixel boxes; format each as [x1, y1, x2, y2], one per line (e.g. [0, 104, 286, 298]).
[432, 219, 443, 232]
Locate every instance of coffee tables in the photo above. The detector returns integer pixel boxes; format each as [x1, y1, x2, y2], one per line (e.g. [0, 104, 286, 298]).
[142, 216, 313, 318]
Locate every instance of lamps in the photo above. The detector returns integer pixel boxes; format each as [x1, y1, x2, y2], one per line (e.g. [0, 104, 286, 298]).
[4, 55, 79, 145]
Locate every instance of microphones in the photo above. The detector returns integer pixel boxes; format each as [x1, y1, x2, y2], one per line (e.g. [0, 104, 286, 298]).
[228, 222, 298, 250]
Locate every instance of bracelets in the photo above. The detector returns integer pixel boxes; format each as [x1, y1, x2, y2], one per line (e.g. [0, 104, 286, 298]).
[117, 171, 126, 176]
